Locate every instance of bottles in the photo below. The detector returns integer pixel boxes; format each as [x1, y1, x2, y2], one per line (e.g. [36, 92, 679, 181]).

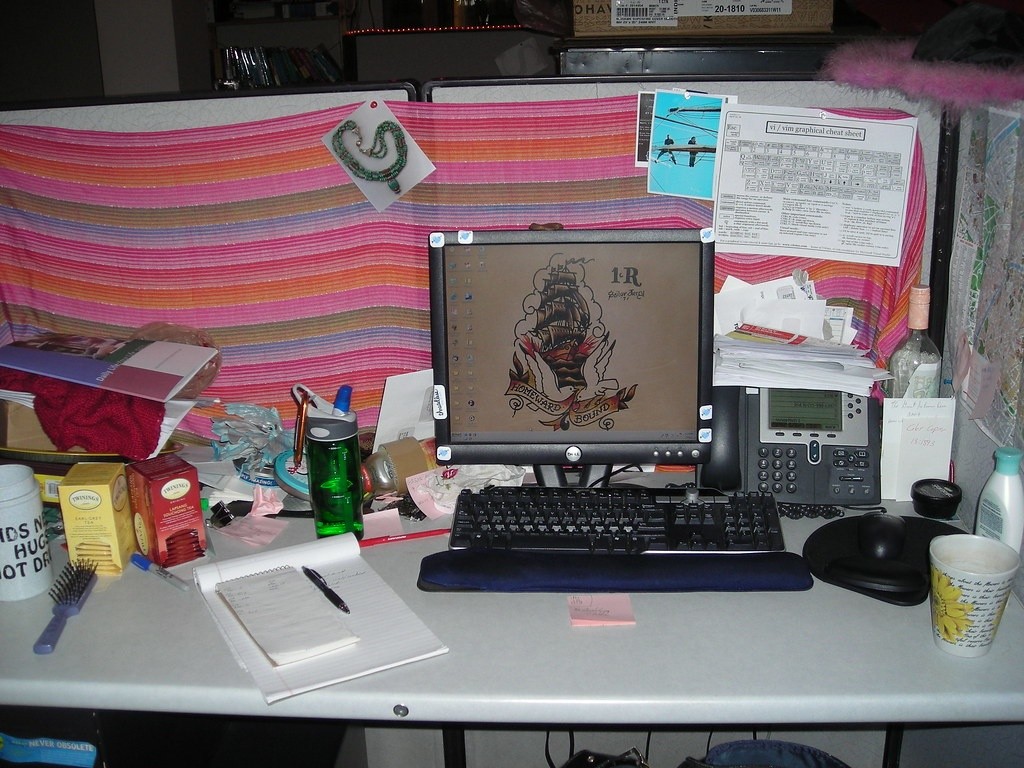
[888, 284, 942, 398]
[292, 381, 365, 543]
[0, 463, 52, 602]
[974, 446, 1024, 553]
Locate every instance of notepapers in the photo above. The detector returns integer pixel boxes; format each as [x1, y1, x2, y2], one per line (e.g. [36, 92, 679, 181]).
[567, 595, 636, 627]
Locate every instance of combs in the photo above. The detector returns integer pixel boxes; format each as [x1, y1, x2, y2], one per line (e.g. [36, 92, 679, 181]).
[34, 559, 99, 655]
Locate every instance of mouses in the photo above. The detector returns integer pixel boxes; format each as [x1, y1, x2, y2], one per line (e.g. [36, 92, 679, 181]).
[858, 513, 906, 561]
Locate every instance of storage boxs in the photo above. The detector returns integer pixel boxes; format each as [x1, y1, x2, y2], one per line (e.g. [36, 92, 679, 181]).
[125, 453, 207, 566]
[0, 400, 87, 456]
[573, 0, 836, 37]
[58, 461, 139, 576]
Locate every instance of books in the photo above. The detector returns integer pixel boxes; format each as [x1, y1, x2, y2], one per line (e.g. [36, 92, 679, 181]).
[0, 331, 219, 461]
[193, 532, 449, 704]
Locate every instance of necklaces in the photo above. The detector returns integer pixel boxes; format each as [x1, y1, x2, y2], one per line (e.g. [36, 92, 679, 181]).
[332, 120, 408, 195]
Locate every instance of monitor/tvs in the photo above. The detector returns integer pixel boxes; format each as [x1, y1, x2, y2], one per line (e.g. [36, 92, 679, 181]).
[427, 228, 716, 492]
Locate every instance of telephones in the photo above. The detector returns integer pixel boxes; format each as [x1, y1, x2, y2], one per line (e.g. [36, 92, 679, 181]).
[702, 386, 880, 505]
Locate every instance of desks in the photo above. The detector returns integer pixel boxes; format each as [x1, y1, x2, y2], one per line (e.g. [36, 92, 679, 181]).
[0, 467, 1024, 768]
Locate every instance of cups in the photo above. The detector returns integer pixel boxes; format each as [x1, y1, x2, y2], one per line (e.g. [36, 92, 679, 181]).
[930, 533, 1021, 657]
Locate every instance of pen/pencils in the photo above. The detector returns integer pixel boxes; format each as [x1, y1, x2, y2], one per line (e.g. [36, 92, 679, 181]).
[302, 564, 352, 616]
[131, 552, 189, 592]
[359, 526, 453, 548]
[293, 391, 309, 467]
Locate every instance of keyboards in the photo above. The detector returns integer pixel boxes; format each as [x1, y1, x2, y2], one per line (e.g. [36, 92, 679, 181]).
[449, 485, 788, 555]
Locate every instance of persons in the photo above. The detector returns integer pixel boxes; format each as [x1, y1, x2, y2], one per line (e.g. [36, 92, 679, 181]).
[7, 340, 117, 361]
[655, 134, 678, 165]
[688, 137, 699, 168]
